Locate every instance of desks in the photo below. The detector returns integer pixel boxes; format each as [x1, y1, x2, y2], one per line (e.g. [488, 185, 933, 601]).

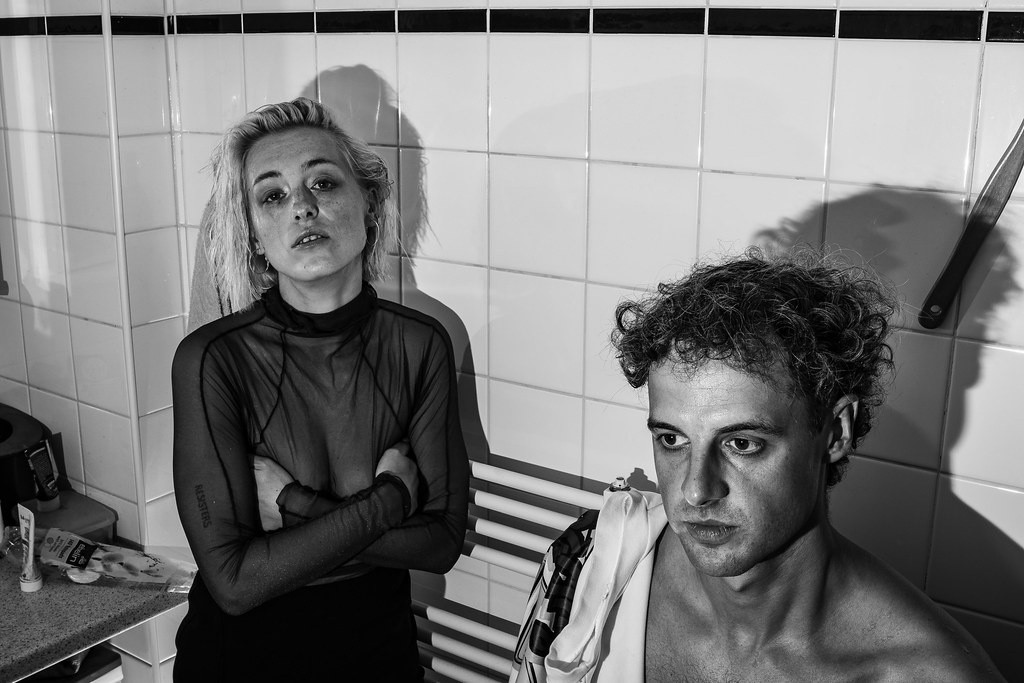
[0, 540, 191, 683]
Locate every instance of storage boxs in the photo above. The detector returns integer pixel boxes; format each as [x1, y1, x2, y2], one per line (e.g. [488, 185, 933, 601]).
[12, 489, 115, 545]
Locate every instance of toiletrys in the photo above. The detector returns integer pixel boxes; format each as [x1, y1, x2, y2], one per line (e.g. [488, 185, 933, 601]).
[22, 438, 62, 513]
[16, 501, 45, 594]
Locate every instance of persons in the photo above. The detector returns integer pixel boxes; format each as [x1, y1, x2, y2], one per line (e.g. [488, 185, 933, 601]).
[510, 242, 1003, 683]
[173, 99, 470, 683]
